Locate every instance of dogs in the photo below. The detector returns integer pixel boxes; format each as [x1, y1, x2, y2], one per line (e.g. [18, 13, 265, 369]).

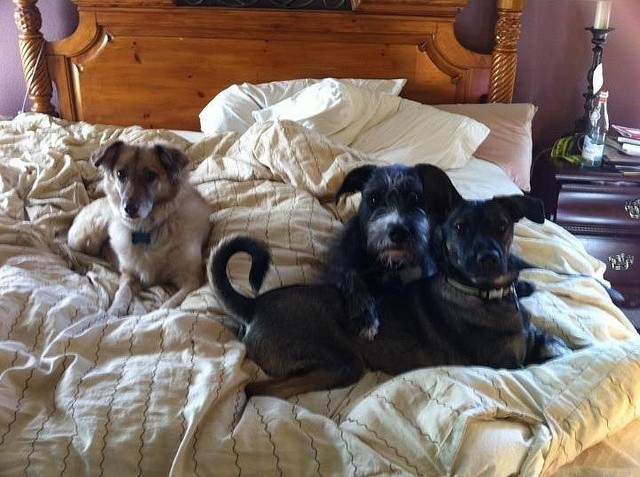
[326, 164, 464, 341]
[66, 139, 211, 317]
[208, 193, 578, 396]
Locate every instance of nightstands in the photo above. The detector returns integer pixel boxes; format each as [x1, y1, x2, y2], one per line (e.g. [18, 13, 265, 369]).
[539, 146, 640, 310]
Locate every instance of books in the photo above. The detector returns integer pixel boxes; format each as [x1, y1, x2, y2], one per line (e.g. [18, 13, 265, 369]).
[603, 130, 640, 176]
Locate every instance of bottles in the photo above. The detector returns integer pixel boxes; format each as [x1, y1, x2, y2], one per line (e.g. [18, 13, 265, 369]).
[580, 89, 609, 170]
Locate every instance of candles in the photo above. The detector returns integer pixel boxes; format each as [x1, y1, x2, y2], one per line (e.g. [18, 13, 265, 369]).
[593, 1, 612, 29]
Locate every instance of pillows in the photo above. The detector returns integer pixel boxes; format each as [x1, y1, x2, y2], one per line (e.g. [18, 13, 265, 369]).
[197, 76, 408, 140]
[433, 101, 540, 197]
[251, 76, 487, 173]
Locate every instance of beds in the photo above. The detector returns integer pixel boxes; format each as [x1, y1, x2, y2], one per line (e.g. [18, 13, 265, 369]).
[0, 0, 639, 477]
